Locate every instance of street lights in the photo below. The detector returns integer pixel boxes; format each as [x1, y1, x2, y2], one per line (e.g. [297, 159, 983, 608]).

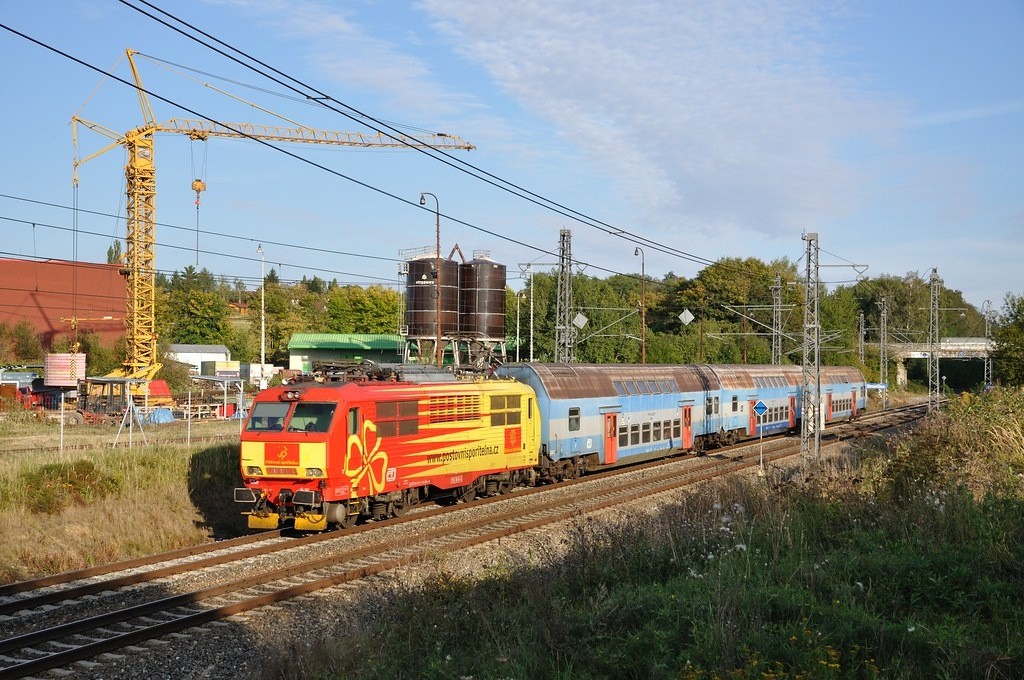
[419, 191, 444, 368]
[255, 244, 268, 388]
[635, 247, 645, 366]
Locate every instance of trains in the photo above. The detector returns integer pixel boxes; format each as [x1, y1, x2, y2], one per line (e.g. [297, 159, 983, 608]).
[235, 359, 867, 536]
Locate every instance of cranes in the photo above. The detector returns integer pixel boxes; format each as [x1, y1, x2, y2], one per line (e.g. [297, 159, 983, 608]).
[70, 48, 477, 400]
